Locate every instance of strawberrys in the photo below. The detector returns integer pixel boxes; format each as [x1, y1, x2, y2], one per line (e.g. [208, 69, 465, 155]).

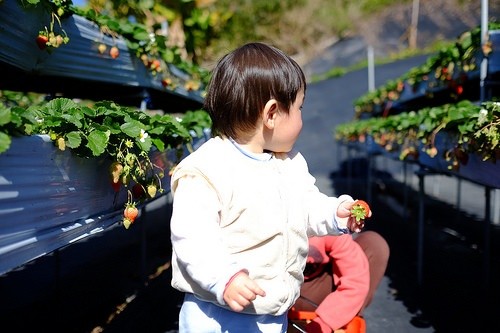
[349, 45, 491, 172]
[38, 7, 201, 230]
[350, 200, 370, 220]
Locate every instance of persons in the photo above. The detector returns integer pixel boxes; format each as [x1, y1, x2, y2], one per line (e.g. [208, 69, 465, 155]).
[169, 41, 389, 332]
